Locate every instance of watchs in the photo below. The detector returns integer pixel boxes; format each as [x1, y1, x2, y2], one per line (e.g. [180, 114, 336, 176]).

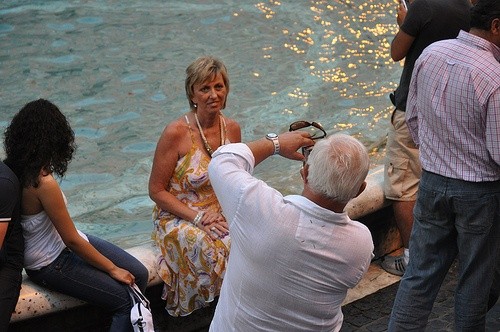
[265, 132, 280, 155]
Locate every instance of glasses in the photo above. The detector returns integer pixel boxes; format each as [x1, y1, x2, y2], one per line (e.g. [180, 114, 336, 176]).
[289, 120, 327, 140]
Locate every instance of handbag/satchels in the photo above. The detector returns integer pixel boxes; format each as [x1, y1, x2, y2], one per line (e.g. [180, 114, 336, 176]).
[127, 283, 156, 332]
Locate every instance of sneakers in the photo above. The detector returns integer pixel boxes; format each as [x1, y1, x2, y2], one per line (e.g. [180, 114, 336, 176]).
[380, 253, 408, 274]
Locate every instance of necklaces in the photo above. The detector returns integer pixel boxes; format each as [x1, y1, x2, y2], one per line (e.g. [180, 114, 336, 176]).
[194, 110, 224, 157]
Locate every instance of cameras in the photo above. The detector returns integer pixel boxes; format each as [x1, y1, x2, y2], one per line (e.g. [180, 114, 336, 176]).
[303, 145, 314, 167]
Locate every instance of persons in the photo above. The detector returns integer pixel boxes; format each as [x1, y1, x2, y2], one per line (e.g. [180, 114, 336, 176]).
[381, 0, 474, 275]
[2, 98, 149, 332]
[388, 0, 500, 332]
[0, 161, 24, 332]
[209, 130, 375, 332]
[148, 56, 241, 317]
